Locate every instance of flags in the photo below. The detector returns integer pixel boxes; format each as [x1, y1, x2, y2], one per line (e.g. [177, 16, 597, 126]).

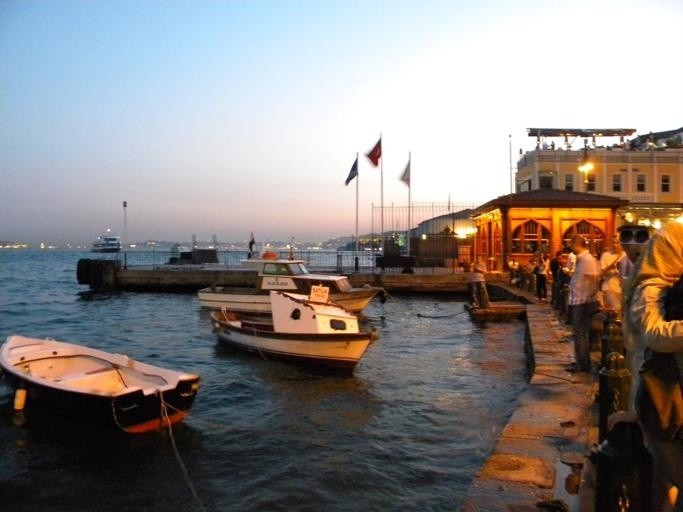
[366, 137, 381, 165]
[400, 160, 410, 186]
[345, 158, 358, 186]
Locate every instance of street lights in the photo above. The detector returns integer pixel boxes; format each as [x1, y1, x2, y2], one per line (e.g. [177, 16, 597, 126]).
[578, 162, 594, 194]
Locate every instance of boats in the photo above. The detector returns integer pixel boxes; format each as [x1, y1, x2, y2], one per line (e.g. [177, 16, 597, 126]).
[170, 244, 190, 257]
[208, 284, 380, 371]
[1, 334, 202, 439]
[196, 242, 393, 318]
[92, 237, 122, 252]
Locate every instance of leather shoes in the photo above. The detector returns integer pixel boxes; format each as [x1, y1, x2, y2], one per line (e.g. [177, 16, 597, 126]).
[565, 362, 591, 372]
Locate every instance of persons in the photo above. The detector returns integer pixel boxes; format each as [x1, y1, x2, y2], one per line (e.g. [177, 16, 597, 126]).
[470, 254, 492, 307]
[607, 220, 683, 512]
[508, 247, 576, 309]
[599, 232, 626, 310]
[566, 236, 598, 371]
